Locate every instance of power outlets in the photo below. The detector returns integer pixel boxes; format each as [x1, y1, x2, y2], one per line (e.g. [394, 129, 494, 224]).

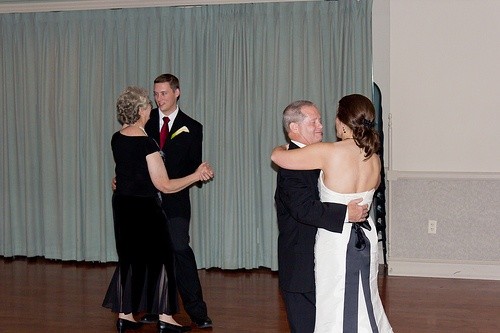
[428, 221, 437, 234]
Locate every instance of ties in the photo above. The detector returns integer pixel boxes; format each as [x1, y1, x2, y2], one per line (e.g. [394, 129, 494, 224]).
[159, 117, 170, 150]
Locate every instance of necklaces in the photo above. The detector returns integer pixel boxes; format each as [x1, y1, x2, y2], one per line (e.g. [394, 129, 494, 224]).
[344, 138, 356, 140]
[140, 126, 148, 135]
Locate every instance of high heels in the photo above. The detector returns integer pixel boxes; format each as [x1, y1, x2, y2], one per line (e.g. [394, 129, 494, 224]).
[116, 318, 143, 333]
[157, 320, 192, 333]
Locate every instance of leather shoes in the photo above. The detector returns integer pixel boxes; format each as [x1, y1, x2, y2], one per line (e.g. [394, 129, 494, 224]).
[141, 312, 158, 323]
[196, 317, 212, 329]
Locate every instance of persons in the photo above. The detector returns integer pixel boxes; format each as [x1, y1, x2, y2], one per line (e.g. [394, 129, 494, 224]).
[273, 100, 370, 333]
[270, 93, 393, 333]
[112, 73, 214, 329]
[99, 85, 215, 333]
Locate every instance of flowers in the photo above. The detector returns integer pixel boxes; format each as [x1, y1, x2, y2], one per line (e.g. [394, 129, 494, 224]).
[171, 126, 190, 138]
